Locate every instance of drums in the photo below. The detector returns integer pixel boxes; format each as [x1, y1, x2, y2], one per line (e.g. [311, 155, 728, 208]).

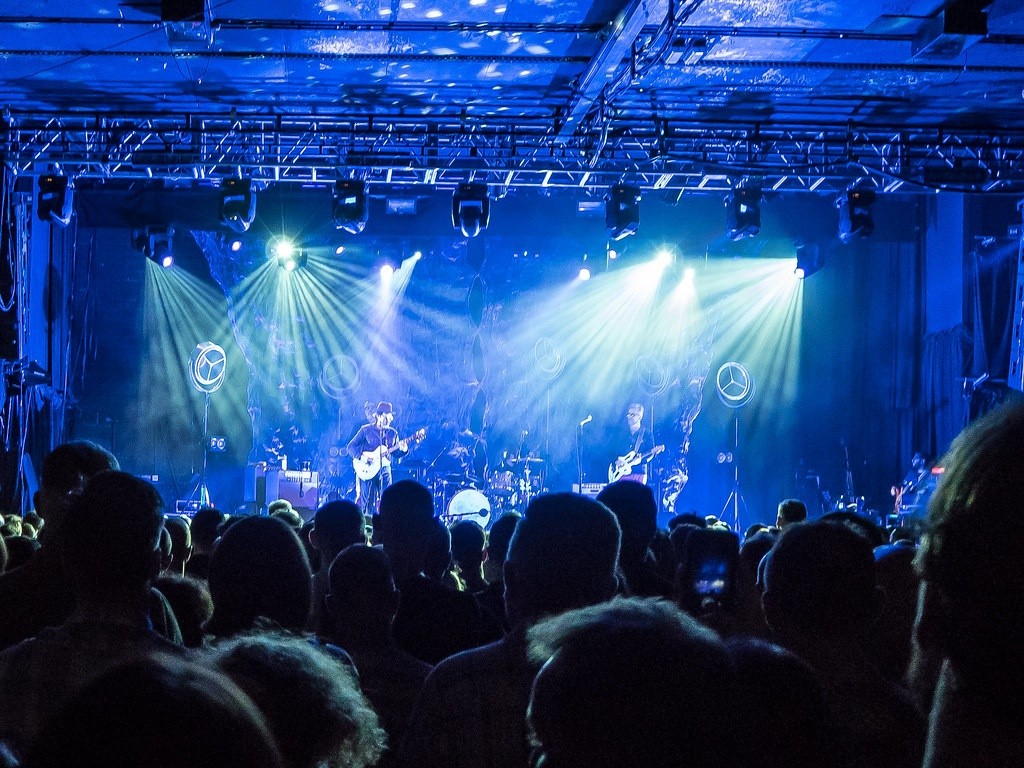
[445, 488, 495, 532]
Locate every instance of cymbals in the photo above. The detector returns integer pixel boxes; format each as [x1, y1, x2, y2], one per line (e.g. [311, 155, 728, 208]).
[512, 457, 543, 462]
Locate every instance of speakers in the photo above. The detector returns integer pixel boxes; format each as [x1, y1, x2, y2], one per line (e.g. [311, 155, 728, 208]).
[244, 464, 319, 511]
[572, 483, 607, 500]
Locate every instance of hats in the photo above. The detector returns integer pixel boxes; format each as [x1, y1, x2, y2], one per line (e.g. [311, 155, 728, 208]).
[373, 402, 396, 414]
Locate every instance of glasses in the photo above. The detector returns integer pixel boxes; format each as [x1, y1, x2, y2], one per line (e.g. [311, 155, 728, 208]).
[628, 412, 639, 417]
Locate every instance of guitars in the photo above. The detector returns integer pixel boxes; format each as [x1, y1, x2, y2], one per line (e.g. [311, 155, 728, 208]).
[352, 427, 428, 482]
[607, 443, 666, 484]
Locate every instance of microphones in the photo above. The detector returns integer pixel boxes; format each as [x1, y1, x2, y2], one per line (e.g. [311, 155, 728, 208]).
[300, 482, 304, 497]
[579, 415, 592, 425]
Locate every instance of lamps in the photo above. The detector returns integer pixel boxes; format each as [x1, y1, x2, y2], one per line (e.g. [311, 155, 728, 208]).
[132, 221, 173, 267]
[726, 189, 762, 241]
[839, 192, 874, 243]
[451, 182, 491, 238]
[603, 182, 641, 241]
[331, 179, 368, 233]
[217, 178, 256, 233]
[38, 175, 73, 227]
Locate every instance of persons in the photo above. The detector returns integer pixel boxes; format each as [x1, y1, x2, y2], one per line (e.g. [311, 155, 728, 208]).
[0, 394, 1024, 768]
